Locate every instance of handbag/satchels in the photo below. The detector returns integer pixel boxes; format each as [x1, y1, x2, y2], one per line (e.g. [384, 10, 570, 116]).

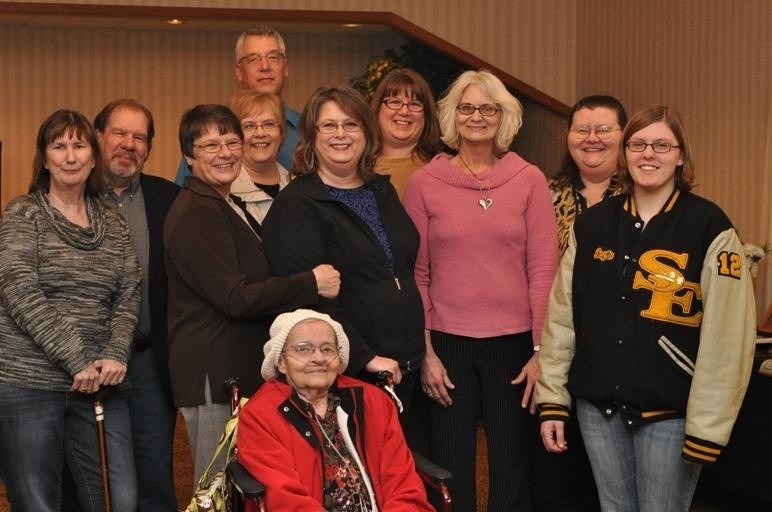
[184, 396, 250, 511]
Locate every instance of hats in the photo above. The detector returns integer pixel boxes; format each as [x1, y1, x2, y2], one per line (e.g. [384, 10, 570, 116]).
[260, 309, 350, 382]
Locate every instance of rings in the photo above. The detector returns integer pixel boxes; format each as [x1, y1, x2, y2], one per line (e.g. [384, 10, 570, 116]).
[78, 385, 89, 391]
[112, 376, 119, 383]
[426, 390, 433, 395]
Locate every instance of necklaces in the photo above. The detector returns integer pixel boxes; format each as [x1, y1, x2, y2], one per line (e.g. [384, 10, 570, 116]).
[456, 149, 499, 210]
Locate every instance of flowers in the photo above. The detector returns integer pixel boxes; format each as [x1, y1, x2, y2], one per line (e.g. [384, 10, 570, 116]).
[350, 56, 401, 101]
[742, 241, 768, 277]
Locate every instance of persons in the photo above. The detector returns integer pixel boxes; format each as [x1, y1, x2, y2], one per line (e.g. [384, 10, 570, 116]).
[258, 83, 426, 454]
[172, 23, 317, 191]
[162, 102, 342, 498]
[368, 68, 457, 205]
[235, 308, 436, 512]
[535, 103, 757, 512]
[0, 108, 143, 512]
[225, 88, 300, 242]
[546, 94, 633, 511]
[403, 70, 561, 511]
[61, 98, 183, 512]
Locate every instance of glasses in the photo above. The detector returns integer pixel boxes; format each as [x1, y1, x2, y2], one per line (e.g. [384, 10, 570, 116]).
[314, 118, 365, 133]
[238, 52, 284, 61]
[193, 139, 242, 152]
[568, 126, 621, 136]
[456, 103, 501, 117]
[240, 123, 282, 132]
[624, 141, 680, 153]
[382, 98, 424, 112]
[282, 342, 341, 355]
